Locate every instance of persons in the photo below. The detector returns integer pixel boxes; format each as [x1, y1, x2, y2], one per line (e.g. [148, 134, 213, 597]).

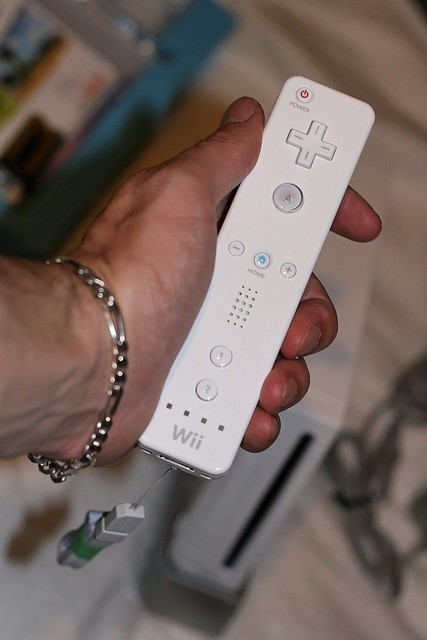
[1, 97, 382, 468]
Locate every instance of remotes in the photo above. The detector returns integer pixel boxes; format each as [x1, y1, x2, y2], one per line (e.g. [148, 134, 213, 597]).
[136, 75, 377, 481]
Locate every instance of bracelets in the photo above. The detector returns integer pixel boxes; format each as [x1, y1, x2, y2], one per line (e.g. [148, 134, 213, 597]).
[26, 257, 128, 484]
